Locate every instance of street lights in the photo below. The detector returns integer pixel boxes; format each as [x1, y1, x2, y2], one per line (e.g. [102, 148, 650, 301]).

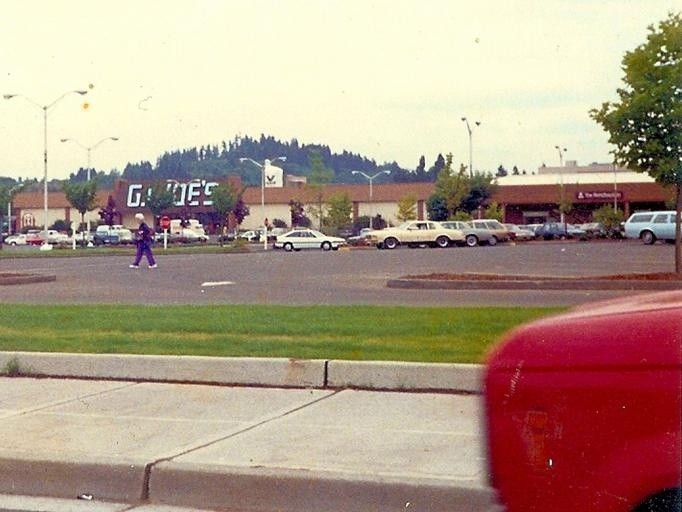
[3, 183, 25, 235]
[238, 157, 287, 250]
[59, 136, 119, 248]
[1, 89, 88, 251]
[351, 169, 392, 230]
[461, 116, 481, 178]
[554, 143, 568, 223]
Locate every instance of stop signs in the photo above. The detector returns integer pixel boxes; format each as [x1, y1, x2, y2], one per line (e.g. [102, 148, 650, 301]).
[159, 216, 170, 228]
[265, 218, 269, 226]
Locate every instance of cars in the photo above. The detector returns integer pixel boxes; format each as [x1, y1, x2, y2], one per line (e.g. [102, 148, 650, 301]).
[348, 218, 624, 250]
[0, 219, 286, 249]
[272, 229, 346, 252]
[481, 288, 682, 511]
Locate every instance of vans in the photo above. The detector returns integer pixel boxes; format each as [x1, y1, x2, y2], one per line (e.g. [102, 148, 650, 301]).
[625, 211, 677, 246]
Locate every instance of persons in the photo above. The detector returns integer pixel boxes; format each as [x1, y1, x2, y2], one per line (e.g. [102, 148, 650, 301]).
[129, 213, 158, 268]
